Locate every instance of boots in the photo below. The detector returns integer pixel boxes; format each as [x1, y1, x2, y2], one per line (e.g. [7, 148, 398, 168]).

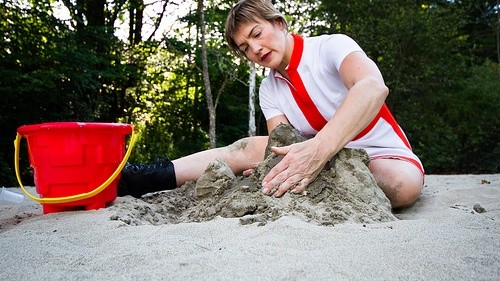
[114, 159, 180, 200]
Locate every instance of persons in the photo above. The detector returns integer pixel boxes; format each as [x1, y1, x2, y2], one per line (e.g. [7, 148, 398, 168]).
[113, 0, 425, 207]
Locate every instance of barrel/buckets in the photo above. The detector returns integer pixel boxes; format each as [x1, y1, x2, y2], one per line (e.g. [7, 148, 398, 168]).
[11, 124, 140, 214]
